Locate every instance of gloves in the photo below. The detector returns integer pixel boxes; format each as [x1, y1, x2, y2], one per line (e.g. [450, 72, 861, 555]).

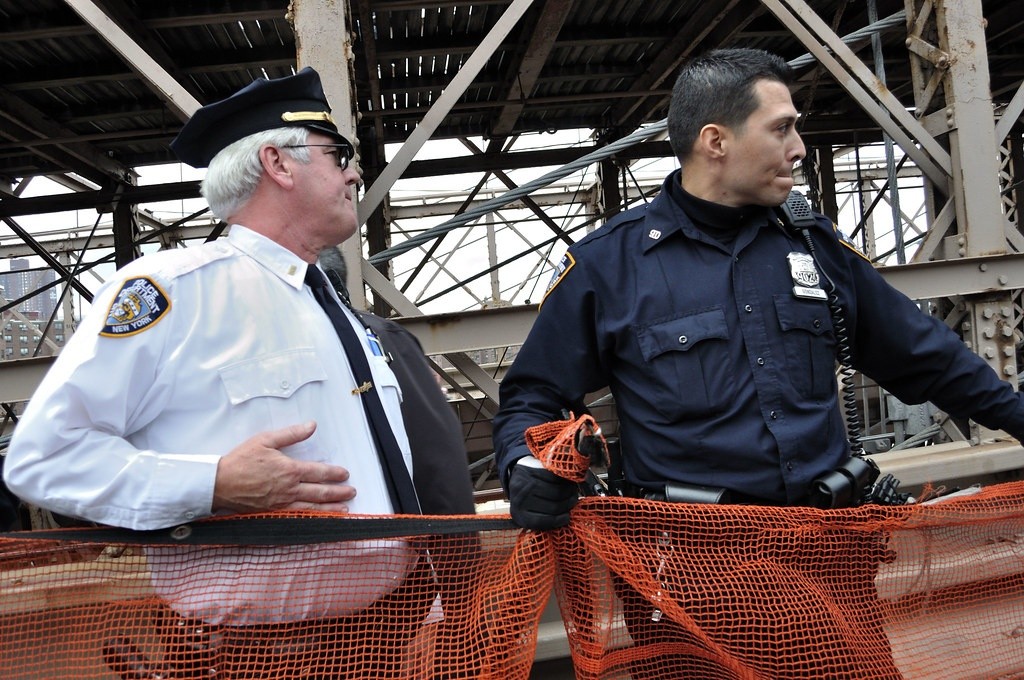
[505, 456, 579, 530]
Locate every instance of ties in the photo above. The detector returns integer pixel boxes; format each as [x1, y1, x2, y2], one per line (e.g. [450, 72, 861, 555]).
[303, 264, 429, 557]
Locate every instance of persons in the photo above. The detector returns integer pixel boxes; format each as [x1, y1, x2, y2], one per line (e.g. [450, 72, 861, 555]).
[494, 48, 1024, 680]
[2, 66, 481, 680]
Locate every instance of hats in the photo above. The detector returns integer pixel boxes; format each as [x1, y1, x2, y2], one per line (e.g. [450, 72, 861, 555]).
[168, 66, 355, 161]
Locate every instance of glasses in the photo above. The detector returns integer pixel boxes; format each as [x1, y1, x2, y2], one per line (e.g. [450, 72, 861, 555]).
[279, 144, 349, 170]
